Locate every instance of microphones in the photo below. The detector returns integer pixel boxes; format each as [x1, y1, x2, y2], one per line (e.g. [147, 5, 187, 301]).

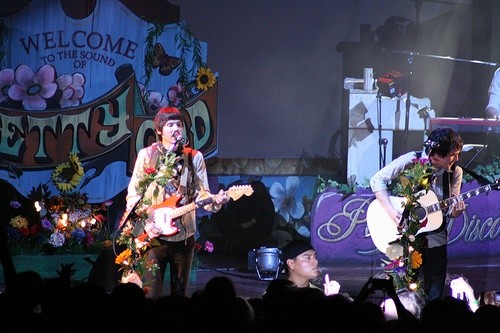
[424, 140, 440, 147]
[173, 131, 183, 142]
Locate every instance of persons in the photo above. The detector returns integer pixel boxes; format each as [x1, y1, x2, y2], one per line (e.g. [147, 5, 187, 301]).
[370, 127, 468, 302]
[127, 108, 230, 298]
[0, 239, 500, 333]
[485, 68, 500, 131]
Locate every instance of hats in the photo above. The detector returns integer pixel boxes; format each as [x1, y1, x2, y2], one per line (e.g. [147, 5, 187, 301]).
[281, 240, 314, 262]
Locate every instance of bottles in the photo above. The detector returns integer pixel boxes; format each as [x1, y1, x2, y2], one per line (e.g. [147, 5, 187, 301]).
[364, 67, 374, 90]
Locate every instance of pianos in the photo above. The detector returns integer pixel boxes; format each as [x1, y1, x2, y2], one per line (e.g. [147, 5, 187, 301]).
[427, 115, 500, 169]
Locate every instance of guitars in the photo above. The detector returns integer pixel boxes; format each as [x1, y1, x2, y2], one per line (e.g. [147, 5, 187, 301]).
[366, 177, 500, 255]
[139, 184, 255, 241]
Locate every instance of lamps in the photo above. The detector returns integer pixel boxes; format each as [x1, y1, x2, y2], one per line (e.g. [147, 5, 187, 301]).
[253, 246, 282, 281]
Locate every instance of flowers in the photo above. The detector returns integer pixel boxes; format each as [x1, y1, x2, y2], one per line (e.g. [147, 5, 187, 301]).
[386, 158, 436, 300]
[9, 152, 214, 290]
[194, 66, 217, 91]
